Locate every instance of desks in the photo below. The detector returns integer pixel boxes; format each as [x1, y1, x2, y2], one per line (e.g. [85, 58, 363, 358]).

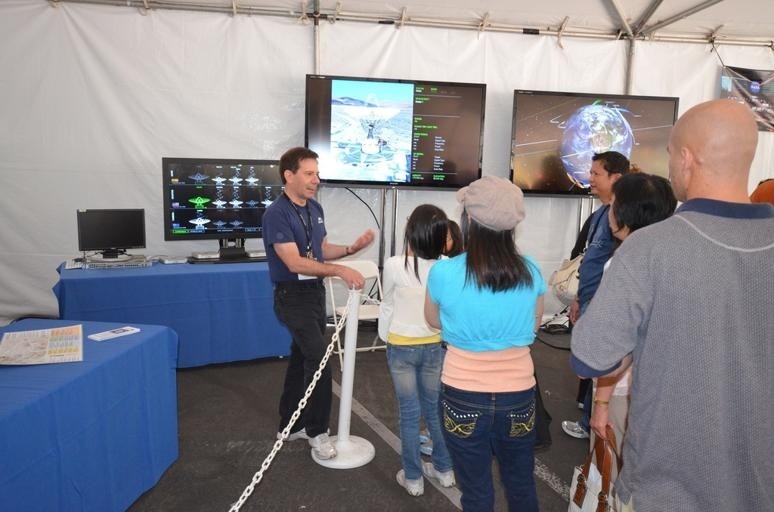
[0, 318, 179, 512]
[52, 249, 293, 369]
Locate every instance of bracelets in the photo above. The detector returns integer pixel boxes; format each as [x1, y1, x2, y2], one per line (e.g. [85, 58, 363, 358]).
[593, 396, 610, 408]
[345, 245, 352, 256]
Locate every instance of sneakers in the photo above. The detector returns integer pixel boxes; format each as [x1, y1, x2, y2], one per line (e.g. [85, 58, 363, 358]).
[276, 425, 330, 441]
[562, 420, 591, 439]
[396, 468, 424, 497]
[423, 463, 456, 487]
[306, 432, 337, 460]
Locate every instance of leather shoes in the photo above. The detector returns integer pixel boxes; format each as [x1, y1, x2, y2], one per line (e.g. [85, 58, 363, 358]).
[419, 428, 433, 456]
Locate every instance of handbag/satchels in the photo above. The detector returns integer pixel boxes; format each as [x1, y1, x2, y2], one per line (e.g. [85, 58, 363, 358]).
[567, 462, 622, 512]
[553, 253, 586, 307]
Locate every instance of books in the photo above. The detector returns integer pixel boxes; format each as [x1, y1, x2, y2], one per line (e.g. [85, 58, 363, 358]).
[87, 325, 141, 343]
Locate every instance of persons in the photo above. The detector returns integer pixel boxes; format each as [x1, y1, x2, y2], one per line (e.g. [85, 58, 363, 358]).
[424, 175, 553, 512]
[566, 171, 678, 511]
[567, 98, 772, 512]
[554, 150, 631, 441]
[260, 146, 376, 462]
[439, 219, 462, 259]
[532, 371, 553, 455]
[378, 204, 461, 498]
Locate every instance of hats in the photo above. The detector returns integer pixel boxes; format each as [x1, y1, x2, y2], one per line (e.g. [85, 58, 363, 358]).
[456, 175, 527, 232]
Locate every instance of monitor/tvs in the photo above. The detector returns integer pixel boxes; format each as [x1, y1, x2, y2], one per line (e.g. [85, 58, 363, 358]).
[509, 89, 680, 200]
[77, 209, 146, 262]
[304, 72, 486, 192]
[162, 157, 286, 264]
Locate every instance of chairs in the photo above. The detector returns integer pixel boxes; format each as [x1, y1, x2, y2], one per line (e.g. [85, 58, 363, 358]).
[326, 259, 388, 372]
